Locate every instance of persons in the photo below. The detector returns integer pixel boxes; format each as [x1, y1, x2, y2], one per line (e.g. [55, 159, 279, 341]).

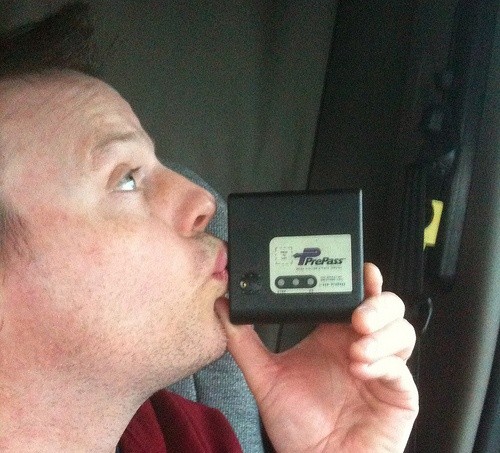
[1, 49, 420, 453]
